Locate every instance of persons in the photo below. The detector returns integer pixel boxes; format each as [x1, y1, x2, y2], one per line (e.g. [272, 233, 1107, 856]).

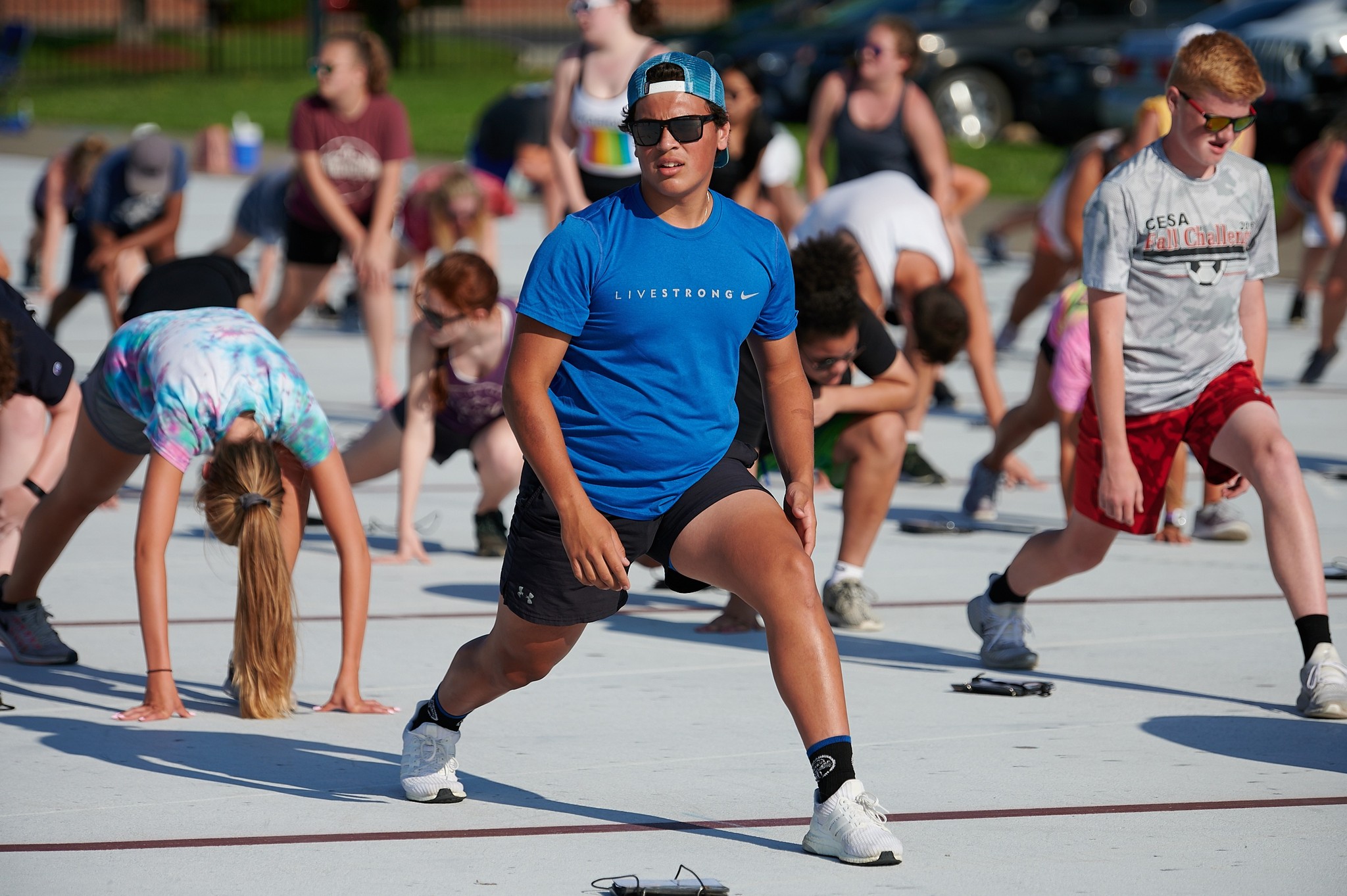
[399, 48, 907, 868]
[0, 0, 1347, 635]
[0, 307, 400, 723]
[968, 30, 1347, 719]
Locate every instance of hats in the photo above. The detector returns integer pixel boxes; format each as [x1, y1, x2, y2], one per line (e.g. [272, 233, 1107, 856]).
[627, 51, 729, 169]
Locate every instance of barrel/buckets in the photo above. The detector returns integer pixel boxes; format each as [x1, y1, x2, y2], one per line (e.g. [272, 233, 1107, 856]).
[231, 110, 264, 172]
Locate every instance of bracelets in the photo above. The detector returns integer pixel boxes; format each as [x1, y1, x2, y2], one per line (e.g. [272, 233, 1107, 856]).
[146, 669, 172, 674]
[24, 479, 47, 497]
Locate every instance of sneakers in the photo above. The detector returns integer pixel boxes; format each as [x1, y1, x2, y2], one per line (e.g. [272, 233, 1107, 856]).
[1192, 497, 1253, 542]
[966, 573, 1039, 671]
[802, 778, 905, 867]
[399, 700, 467, 804]
[961, 486, 998, 521]
[475, 507, 509, 558]
[1297, 643, 1347, 719]
[820, 576, 879, 633]
[0, 572, 79, 665]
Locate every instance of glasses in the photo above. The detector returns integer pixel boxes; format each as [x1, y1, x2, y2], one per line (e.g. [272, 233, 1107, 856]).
[629, 113, 714, 148]
[568, 0, 613, 14]
[307, 56, 361, 74]
[720, 86, 755, 102]
[1177, 89, 1259, 134]
[861, 39, 907, 60]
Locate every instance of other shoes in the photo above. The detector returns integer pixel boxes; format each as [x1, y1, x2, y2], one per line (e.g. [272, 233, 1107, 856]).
[932, 378, 955, 410]
[306, 299, 336, 320]
[896, 442, 945, 485]
